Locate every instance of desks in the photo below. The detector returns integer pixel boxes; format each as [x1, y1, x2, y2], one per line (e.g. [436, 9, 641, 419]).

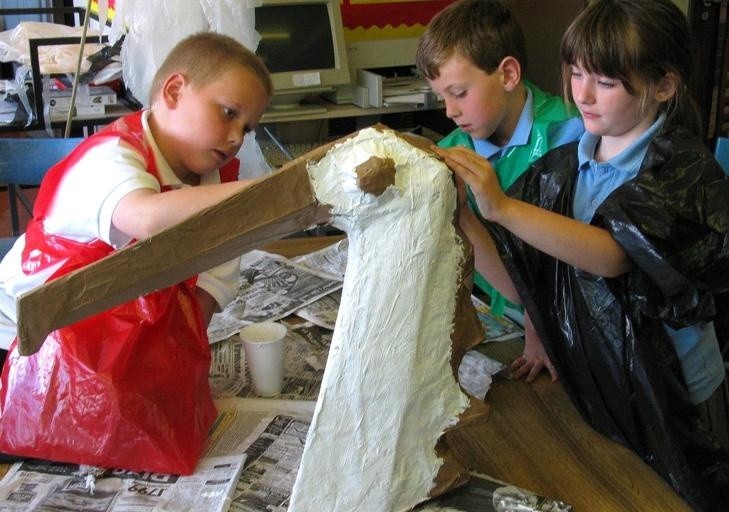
[251, 99, 446, 166]
[0, 234, 693, 512]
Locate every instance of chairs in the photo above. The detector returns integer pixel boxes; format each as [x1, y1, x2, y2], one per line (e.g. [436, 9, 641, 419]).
[0, 138, 87, 264]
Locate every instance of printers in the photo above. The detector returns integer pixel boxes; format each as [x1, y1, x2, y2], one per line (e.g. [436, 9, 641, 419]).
[320, 38, 436, 110]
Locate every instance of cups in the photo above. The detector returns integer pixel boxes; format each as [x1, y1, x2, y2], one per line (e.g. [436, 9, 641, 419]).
[240, 322, 289, 397]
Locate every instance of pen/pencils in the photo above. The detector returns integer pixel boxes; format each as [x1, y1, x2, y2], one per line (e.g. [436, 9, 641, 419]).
[263, 126, 293, 160]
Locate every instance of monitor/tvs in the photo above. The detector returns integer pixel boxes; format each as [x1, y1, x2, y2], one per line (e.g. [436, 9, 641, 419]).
[255, 1, 352, 118]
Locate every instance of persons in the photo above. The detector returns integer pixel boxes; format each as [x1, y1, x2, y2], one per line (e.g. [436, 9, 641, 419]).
[1, 32, 274, 436]
[418, 11, 579, 389]
[430, 0, 729, 512]
